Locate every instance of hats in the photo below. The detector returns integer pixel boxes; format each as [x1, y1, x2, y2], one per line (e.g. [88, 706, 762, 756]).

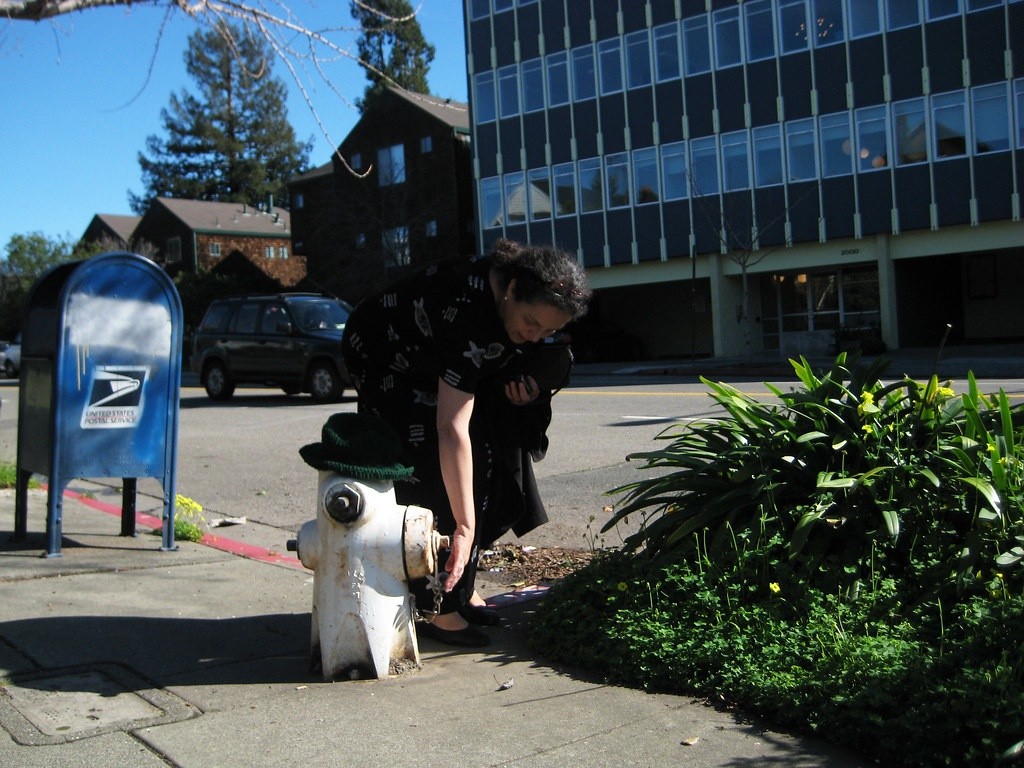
[299, 413, 415, 479]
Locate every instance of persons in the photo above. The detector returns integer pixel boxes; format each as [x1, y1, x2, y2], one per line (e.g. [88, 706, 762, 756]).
[342, 239, 587, 648]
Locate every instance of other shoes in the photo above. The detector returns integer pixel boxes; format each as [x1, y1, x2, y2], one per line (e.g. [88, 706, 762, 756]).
[415, 612, 491, 648]
[457, 601, 500, 626]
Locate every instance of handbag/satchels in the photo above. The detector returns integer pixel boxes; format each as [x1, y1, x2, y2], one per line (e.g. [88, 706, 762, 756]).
[506, 345, 575, 388]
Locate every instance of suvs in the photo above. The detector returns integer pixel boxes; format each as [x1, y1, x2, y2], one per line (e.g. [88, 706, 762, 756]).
[192, 292, 358, 405]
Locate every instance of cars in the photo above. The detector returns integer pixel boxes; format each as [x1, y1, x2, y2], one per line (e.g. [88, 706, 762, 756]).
[0, 331, 21, 379]
